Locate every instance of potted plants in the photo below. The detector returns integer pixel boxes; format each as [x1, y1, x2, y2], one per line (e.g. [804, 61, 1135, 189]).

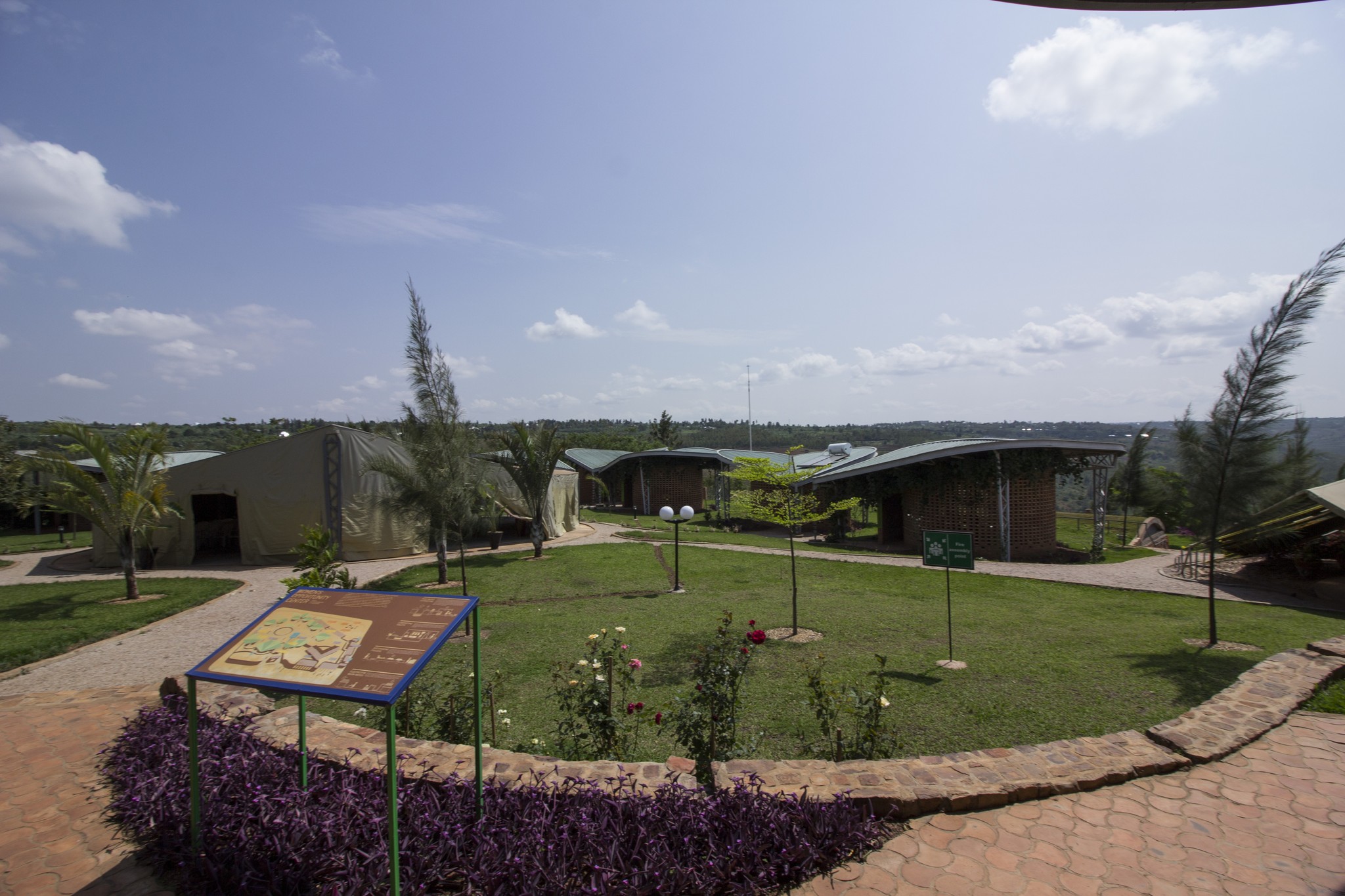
[475, 480, 508, 550]
[135, 527, 159, 571]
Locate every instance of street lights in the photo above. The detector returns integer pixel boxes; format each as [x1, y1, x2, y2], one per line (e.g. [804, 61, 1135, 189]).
[659, 505, 694, 590]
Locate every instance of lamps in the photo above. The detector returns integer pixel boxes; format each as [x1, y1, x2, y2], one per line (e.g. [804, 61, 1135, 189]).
[57, 526, 65, 532]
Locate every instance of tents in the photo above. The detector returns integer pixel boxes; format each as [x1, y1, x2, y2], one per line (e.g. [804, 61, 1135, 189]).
[93, 423, 581, 569]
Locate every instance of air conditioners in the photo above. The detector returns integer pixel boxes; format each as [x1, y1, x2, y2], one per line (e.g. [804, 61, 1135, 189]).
[633, 506, 636, 510]
